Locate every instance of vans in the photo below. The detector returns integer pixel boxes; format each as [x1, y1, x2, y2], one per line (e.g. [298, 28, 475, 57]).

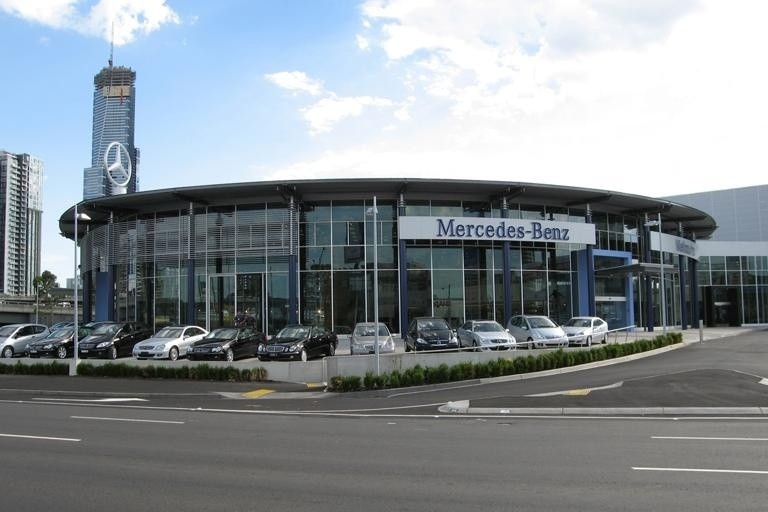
[506, 315, 569, 348]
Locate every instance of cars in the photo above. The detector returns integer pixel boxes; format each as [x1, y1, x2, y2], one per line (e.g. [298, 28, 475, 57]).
[128, 324, 209, 363]
[185, 327, 265, 362]
[400, 316, 459, 352]
[454, 319, 516, 351]
[559, 316, 608, 347]
[193, 305, 326, 326]
[0, 319, 143, 362]
[257, 325, 338, 361]
[345, 321, 394, 355]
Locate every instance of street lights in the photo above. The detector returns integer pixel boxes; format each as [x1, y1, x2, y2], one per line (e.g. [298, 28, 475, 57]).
[364, 195, 380, 378]
[316, 244, 328, 270]
[644, 218, 667, 339]
[213, 207, 233, 326]
[68, 199, 96, 365]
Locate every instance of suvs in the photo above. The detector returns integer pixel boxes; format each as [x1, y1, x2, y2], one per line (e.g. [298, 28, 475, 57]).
[57, 301, 70, 309]
